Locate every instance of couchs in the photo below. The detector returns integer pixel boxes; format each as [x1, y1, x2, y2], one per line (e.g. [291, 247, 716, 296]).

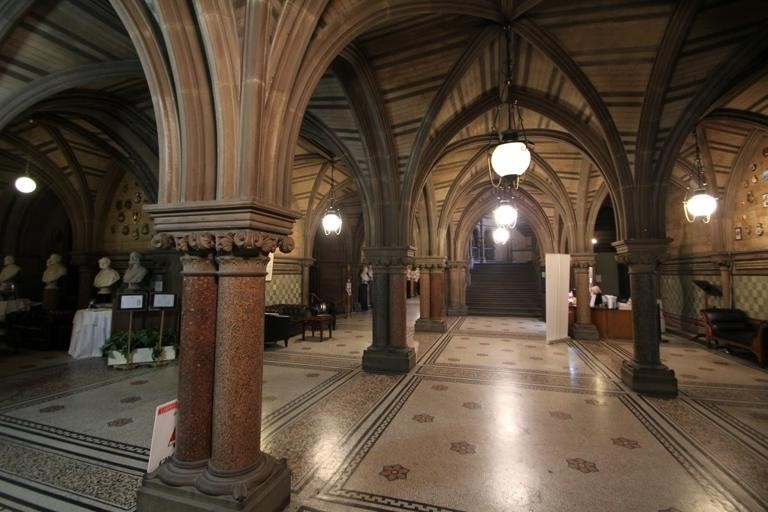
[264, 303, 307, 347]
[701, 306, 768, 367]
[4, 305, 74, 350]
[311, 301, 337, 328]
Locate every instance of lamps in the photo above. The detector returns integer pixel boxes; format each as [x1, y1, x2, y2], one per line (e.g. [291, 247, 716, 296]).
[320, 156, 344, 236]
[487, 24, 535, 247]
[683, 125, 723, 226]
[14, 117, 39, 195]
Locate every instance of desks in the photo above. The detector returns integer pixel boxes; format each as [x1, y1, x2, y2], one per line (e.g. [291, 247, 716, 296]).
[69, 304, 113, 362]
[0, 297, 32, 344]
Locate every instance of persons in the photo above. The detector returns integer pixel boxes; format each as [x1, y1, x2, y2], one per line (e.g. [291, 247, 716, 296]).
[123, 251, 147, 289]
[92, 257, 121, 294]
[42, 253, 67, 289]
[360, 265, 421, 283]
[0, 255, 22, 283]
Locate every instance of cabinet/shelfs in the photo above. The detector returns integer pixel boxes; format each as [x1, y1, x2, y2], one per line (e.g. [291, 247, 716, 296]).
[568, 301, 632, 341]
